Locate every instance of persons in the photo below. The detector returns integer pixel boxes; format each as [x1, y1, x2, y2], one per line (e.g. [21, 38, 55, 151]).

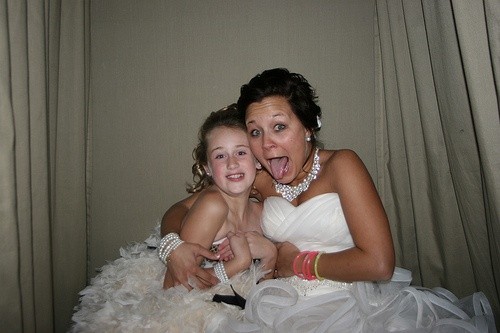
[68, 101, 279, 333]
[157, 69, 498, 333]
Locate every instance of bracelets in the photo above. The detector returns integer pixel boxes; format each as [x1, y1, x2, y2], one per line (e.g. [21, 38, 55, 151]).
[159, 233, 184, 266]
[213, 260, 229, 284]
[293, 250, 325, 281]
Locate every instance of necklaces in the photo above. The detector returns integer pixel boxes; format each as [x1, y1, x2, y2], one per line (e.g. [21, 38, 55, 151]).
[270, 147, 321, 202]
